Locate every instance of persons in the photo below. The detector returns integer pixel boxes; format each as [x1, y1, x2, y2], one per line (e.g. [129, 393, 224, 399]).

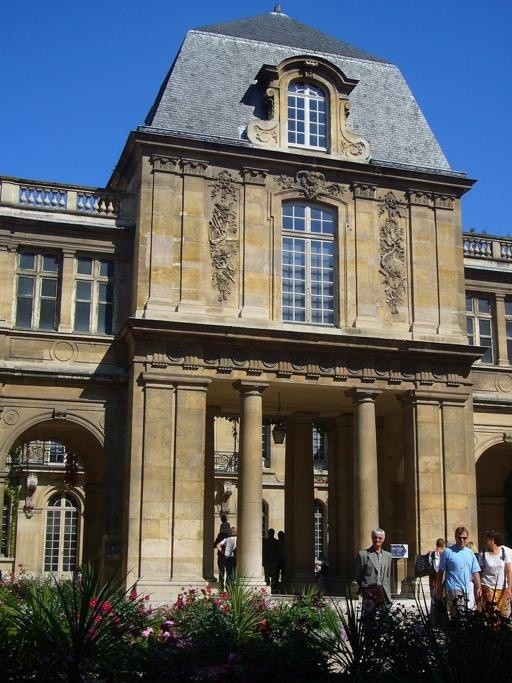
[428, 537, 449, 625]
[478, 529, 512, 627]
[262, 528, 279, 590]
[435, 526, 483, 630]
[277, 530, 285, 582]
[466, 541, 481, 553]
[219, 515, 230, 532]
[352, 527, 393, 618]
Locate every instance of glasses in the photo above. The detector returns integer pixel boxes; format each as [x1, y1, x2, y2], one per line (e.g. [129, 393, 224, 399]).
[373, 537, 383, 540]
[455, 536, 468, 540]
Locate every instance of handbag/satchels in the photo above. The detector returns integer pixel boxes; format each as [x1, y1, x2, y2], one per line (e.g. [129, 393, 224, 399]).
[496, 588, 511, 618]
[416, 556, 432, 578]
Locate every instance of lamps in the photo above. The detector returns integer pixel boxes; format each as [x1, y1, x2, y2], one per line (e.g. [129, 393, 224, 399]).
[272, 390, 287, 443]
